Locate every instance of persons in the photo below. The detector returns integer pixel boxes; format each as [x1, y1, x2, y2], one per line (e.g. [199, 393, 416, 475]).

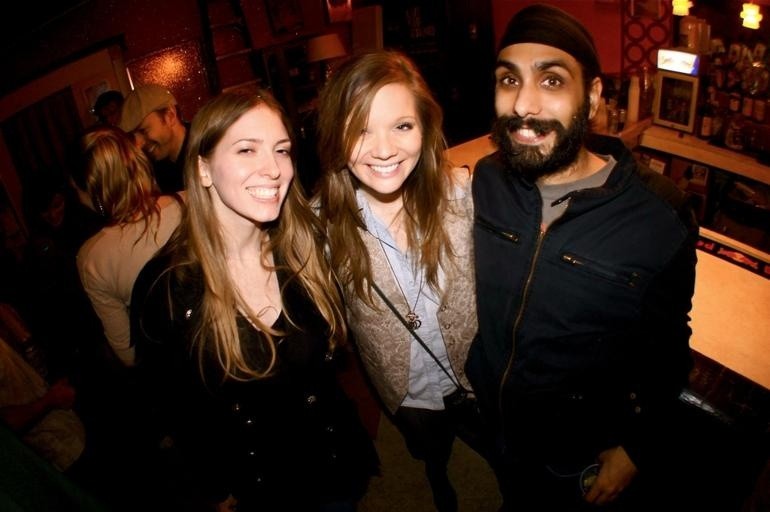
[127, 83, 384, 511]
[1, 300, 108, 510]
[461, 0, 704, 512]
[119, 86, 191, 194]
[69, 131, 191, 367]
[18, 168, 101, 289]
[293, 48, 483, 511]
[95, 91, 125, 128]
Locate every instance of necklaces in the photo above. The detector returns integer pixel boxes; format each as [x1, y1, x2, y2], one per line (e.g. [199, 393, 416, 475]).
[370, 235, 429, 330]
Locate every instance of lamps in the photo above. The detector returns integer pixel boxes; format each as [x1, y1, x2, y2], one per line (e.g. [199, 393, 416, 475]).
[307, 30, 349, 82]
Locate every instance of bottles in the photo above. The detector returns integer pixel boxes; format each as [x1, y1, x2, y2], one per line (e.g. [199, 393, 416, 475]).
[679, 9, 710, 52]
[627, 63, 655, 123]
[699, 40, 768, 156]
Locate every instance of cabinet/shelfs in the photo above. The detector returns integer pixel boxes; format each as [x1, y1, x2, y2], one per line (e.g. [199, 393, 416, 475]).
[211, 16, 306, 94]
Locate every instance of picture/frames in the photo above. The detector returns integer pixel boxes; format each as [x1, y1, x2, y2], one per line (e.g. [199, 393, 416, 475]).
[653, 67, 701, 136]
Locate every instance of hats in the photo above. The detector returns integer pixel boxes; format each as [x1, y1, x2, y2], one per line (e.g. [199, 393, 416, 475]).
[496, 5, 601, 75]
[120, 85, 177, 133]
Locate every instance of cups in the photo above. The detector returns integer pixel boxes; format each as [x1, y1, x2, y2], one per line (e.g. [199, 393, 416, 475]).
[579, 463, 602, 497]
[609, 109, 626, 135]
